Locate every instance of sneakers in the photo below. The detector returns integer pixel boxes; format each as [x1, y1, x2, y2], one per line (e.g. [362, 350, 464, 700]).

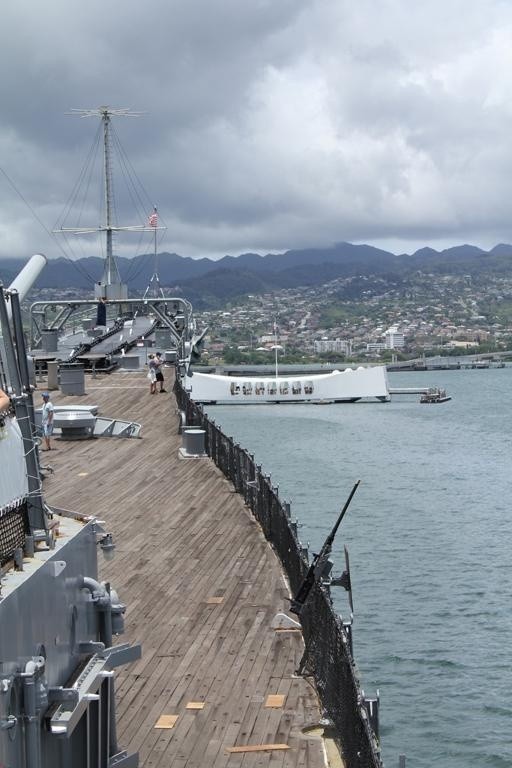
[42, 446, 51, 451]
[151, 389, 167, 394]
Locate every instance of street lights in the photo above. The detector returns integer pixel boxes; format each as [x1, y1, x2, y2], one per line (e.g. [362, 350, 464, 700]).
[271, 327, 282, 382]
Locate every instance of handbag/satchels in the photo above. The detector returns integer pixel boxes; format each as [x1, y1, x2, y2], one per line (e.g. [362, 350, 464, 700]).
[147, 368, 158, 384]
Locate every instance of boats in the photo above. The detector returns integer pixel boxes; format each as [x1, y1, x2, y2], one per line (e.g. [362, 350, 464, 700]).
[418, 386, 454, 405]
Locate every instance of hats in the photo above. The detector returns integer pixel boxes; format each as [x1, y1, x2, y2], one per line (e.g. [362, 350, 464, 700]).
[156, 352, 162, 355]
[42, 393, 49, 398]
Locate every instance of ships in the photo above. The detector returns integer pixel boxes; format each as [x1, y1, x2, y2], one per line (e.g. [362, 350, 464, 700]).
[0, 104, 385, 767]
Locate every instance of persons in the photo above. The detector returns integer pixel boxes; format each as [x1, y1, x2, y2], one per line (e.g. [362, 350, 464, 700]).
[153, 352, 167, 392]
[41, 391, 54, 452]
[148, 353, 162, 394]
